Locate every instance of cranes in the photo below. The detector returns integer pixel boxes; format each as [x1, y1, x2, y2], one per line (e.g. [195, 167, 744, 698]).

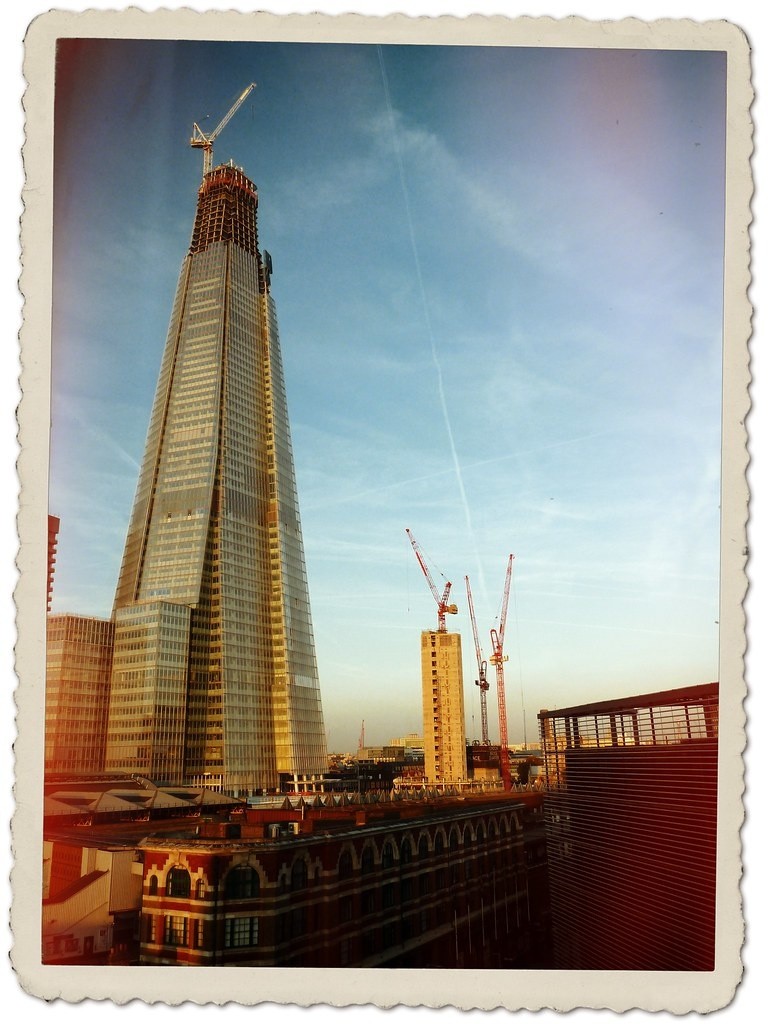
[463, 575, 490, 746]
[405, 528, 457, 633]
[358, 719, 366, 750]
[489, 553, 514, 786]
[189, 82, 257, 174]
